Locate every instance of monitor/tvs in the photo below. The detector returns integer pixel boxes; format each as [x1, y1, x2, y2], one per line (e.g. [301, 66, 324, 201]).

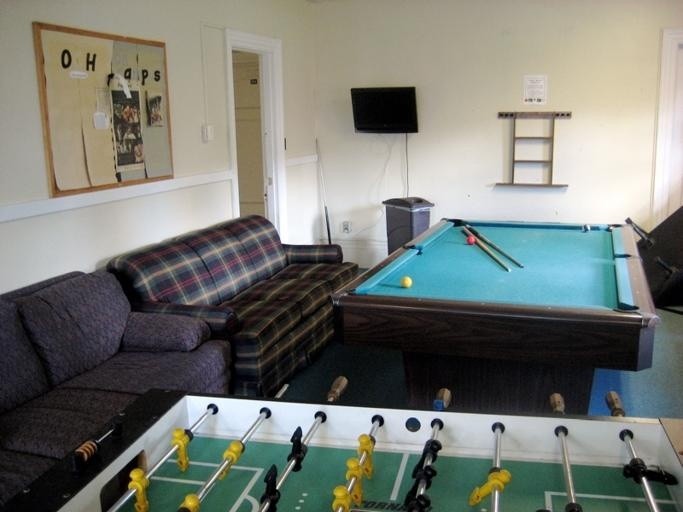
[350, 87, 418, 133]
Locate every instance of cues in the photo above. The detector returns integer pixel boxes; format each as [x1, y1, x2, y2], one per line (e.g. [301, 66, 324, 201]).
[315, 139, 331, 245]
[466, 225, 524, 268]
[463, 227, 510, 272]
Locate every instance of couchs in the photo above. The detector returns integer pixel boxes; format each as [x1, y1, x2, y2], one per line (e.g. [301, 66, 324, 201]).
[107, 215, 359, 400]
[0, 269, 232, 505]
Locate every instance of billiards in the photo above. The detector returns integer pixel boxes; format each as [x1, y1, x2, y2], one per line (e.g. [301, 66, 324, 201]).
[466, 235, 475, 245]
[582, 224, 590, 232]
[400, 276, 411, 287]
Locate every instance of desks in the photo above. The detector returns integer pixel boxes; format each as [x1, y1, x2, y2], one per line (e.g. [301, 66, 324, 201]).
[328, 218, 657, 415]
